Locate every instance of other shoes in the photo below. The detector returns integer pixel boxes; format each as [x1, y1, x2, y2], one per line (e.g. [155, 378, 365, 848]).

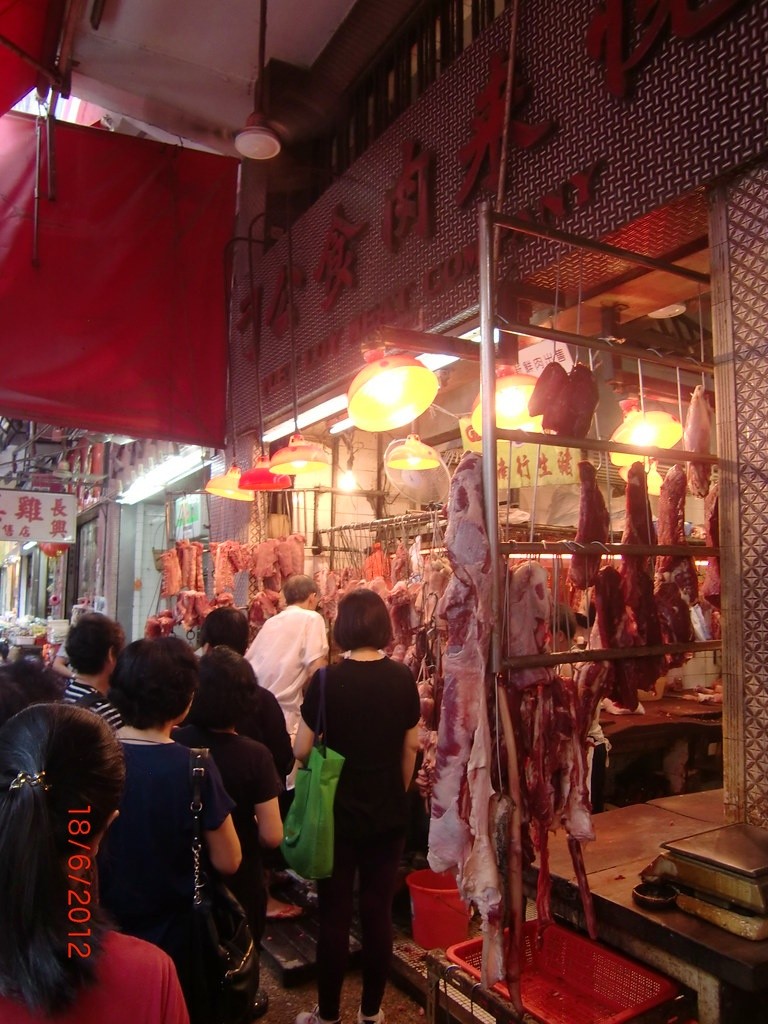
[251, 989, 269, 1020]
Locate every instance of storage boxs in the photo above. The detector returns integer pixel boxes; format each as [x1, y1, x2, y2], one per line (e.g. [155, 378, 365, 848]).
[47, 619, 69, 644]
[447, 919, 678, 1024]
[11, 636, 36, 646]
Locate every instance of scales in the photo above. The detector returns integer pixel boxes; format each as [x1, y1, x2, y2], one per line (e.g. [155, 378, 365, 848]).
[639, 821, 768, 916]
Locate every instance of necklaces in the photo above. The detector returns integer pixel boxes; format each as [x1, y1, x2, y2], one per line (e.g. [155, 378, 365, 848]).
[112, 737, 167, 744]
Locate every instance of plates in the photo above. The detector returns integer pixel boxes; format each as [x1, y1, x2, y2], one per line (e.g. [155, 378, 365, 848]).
[633, 881, 678, 907]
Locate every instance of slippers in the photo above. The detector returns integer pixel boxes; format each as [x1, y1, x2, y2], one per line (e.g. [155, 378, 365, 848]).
[265, 904, 302, 919]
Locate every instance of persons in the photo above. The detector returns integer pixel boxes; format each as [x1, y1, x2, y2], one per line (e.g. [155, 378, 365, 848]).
[0, 574, 420, 1024]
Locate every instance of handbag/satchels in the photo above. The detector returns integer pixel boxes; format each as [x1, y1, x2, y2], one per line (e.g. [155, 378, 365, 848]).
[278, 666, 344, 879]
[167, 868, 259, 1020]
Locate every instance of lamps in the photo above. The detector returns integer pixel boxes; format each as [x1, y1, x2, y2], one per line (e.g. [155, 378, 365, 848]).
[607, 360, 682, 467]
[617, 460, 663, 497]
[471, 366, 543, 442]
[347, 344, 439, 432]
[238, 210, 293, 491]
[233, 111, 290, 160]
[205, 238, 263, 501]
[269, 211, 331, 475]
[386, 419, 440, 470]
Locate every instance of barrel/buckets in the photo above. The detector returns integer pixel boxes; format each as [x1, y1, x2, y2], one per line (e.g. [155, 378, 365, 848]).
[408, 868, 473, 951]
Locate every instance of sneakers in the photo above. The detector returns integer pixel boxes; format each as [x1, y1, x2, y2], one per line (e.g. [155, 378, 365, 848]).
[295, 1003, 342, 1024]
[358, 1005, 384, 1024]
